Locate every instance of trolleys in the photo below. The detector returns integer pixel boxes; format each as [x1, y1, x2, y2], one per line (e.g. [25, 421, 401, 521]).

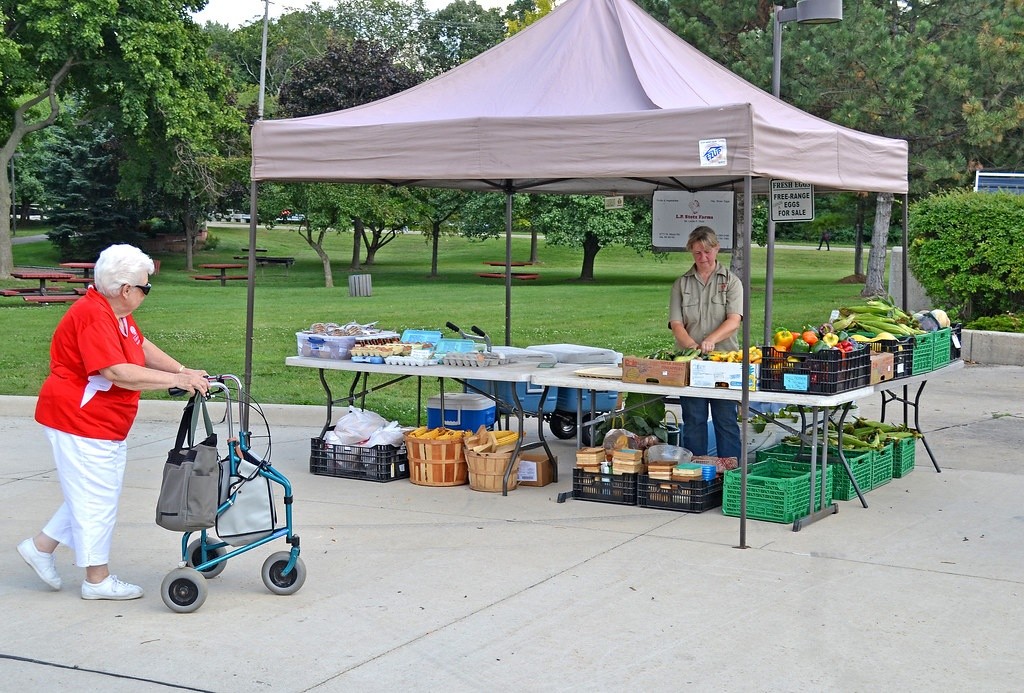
[446, 321, 623, 446]
[161, 375, 306, 614]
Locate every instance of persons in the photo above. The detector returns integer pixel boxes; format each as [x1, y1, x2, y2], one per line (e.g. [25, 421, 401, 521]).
[193, 228, 207, 245]
[18, 244, 212, 601]
[817, 231, 829, 251]
[668, 226, 743, 465]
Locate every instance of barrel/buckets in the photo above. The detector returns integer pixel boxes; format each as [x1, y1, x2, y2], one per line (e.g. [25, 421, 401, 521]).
[737, 408, 860, 464]
[462, 447, 520, 492]
[403, 430, 469, 486]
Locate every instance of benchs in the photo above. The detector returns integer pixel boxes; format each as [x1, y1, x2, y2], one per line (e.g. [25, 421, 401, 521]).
[477, 273, 540, 280]
[0, 269, 94, 305]
[190, 274, 248, 281]
[233, 256, 295, 269]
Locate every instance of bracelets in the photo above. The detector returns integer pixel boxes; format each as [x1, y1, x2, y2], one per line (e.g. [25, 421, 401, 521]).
[178, 365, 185, 374]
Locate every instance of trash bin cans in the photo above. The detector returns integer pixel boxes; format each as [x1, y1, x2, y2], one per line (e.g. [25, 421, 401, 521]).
[349, 274, 372, 297]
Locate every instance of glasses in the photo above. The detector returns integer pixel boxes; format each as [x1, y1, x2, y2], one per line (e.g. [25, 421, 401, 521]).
[123, 282, 152, 295]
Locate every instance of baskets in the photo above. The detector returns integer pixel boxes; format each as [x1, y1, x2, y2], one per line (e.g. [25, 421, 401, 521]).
[572, 468, 636, 505]
[761, 324, 962, 396]
[721, 435, 914, 525]
[636, 474, 722, 513]
[308, 437, 409, 483]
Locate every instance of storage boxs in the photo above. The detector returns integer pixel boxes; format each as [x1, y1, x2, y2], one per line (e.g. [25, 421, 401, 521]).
[309, 437, 410, 483]
[622, 355, 689, 386]
[759, 321, 962, 396]
[572, 459, 722, 513]
[427, 391, 496, 432]
[722, 434, 916, 524]
[657, 410, 717, 457]
[689, 359, 761, 392]
[467, 379, 617, 414]
[517, 454, 557, 487]
[295, 331, 356, 360]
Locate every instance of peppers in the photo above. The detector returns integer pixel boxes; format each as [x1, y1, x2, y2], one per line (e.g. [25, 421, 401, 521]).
[766, 323, 894, 383]
[642, 346, 762, 386]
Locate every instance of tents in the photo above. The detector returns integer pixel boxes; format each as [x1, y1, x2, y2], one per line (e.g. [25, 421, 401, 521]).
[243, 0, 908, 547]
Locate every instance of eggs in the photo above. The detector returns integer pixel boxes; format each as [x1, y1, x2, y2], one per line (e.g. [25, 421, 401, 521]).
[476, 354, 484, 363]
[354, 341, 433, 351]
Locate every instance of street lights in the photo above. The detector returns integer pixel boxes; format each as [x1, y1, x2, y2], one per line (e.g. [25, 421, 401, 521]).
[765, 0, 843, 347]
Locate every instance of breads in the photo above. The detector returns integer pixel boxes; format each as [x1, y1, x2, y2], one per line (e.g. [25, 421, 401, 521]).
[301, 323, 363, 359]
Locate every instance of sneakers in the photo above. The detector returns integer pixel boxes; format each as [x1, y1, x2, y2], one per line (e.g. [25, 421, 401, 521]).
[81, 575, 144, 600]
[17, 538, 61, 590]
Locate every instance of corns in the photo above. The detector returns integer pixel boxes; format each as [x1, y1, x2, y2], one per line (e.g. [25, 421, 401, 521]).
[408, 425, 473, 440]
[790, 419, 914, 448]
[833, 301, 928, 336]
[493, 430, 519, 446]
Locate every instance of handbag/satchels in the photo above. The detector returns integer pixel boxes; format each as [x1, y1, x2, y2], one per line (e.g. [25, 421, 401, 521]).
[154, 390, 222, 532]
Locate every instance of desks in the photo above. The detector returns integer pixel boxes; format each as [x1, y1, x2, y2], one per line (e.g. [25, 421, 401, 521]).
[11, 272, 72, 295]
[483, 261, 533, 266]
[199, 263, 246, 286]
[529, 367, 876, 531]
[242, 247, 270, 252]
[791, 359, 965, 509]
[285, 355, 586, 496]
[60, 262, 95, 288]
